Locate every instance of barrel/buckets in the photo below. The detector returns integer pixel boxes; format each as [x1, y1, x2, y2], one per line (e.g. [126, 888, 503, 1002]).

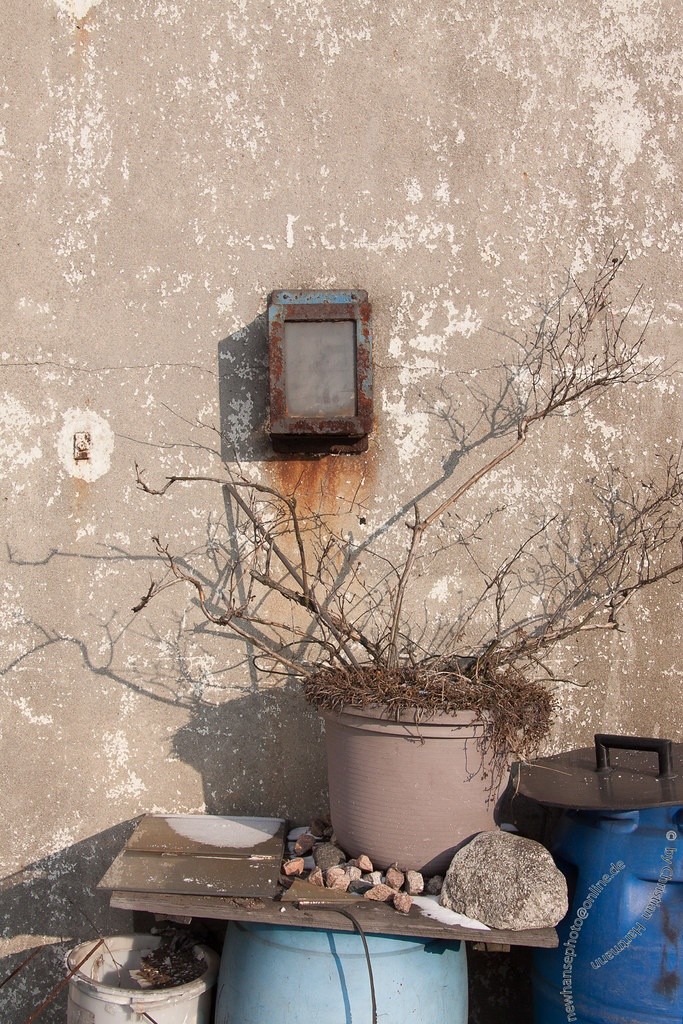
[214, 920, 470, 1023]
[532, 808, 683, 1024]
[65, 934, 219, 1023]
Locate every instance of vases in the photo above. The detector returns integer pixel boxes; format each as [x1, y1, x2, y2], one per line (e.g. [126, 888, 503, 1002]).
[316, 700, 530, 878]
